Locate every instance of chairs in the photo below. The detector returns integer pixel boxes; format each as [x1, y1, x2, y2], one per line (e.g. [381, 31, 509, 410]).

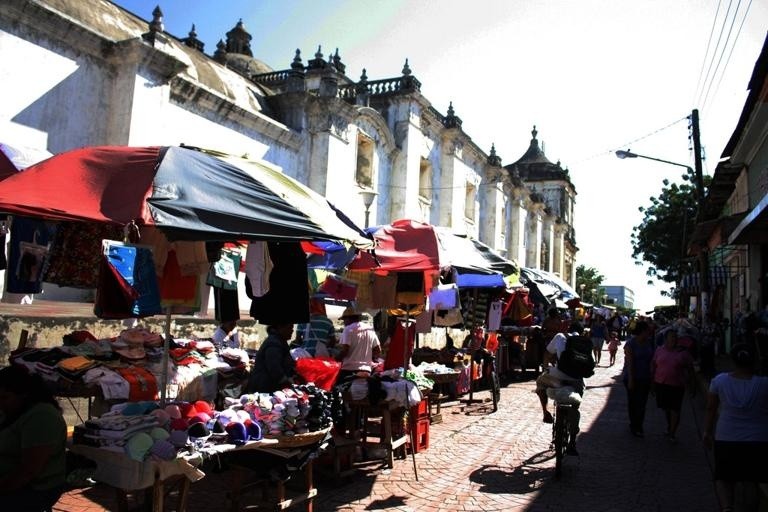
[635, 430, 643, 436]
[544, 411, 552, 421]
[567, 441, 577, 456]
[664, 421, 678, 443]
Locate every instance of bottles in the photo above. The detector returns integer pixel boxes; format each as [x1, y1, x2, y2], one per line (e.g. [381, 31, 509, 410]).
[338, 307, 362, 320]
[611, 331, 618, 337]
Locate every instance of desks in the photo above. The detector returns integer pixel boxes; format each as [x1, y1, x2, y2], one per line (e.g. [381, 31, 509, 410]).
[8, 338, 482, 511]
[613, 147, 712, 343]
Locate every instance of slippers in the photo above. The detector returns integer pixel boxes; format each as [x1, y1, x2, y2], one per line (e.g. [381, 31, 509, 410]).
[556, 332, 595, 379]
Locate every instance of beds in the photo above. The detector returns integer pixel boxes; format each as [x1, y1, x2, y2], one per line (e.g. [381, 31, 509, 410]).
[0, 141, 379, 511]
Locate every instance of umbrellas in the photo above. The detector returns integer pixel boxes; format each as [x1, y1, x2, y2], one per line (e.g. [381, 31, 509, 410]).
[0, 141, 379, 511]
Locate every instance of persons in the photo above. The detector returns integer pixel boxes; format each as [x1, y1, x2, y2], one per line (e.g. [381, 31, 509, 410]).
[733, 299, 765, 344]
[525, 305, 697, 457]
[247, 323, 308, 487]
[702, 342, 768, 511]
[211, 320, 240, 349]
[542, 308, 562, 367]
[536, 311, 585, 423]
[1, 363, 68, 511]
[335, 306, 381, 385]
[296, 299, 336, 359]
